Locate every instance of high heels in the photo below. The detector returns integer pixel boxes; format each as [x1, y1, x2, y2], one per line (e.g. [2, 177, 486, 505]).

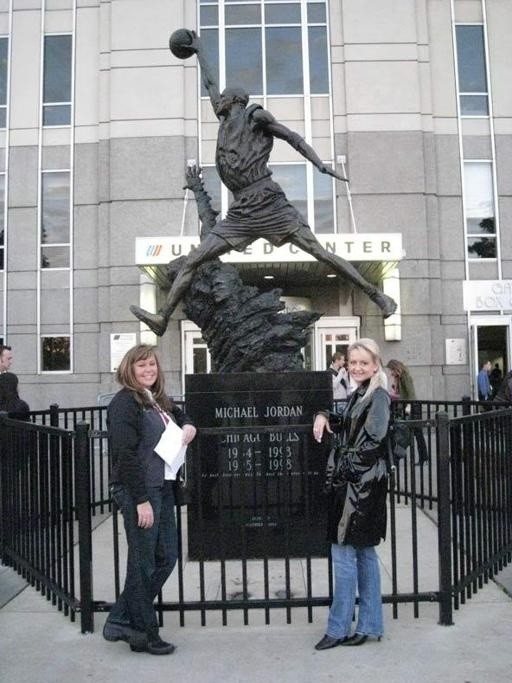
[316, 634, 347, 649]
[341, 633, 380, 646]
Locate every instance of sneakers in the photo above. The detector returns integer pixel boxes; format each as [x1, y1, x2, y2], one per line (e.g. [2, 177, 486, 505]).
[103, 623, 174, 655]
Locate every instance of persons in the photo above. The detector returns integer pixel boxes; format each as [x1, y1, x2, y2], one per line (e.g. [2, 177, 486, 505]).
[476, 359, 494, 431]
[208, 267, 242, 303]
[102, 343, 198, 654]
[162, 162, 220, 332]
[488, 362, 502, 386]
[488, 370, 512, 411]
[129, 26, 397, 337]
[327, 352, 351, 400]
[311, 337, 394, 650]
[386, 358, 428, 466]
[0, 372, 33, 567]
[1, 345, 14, 374]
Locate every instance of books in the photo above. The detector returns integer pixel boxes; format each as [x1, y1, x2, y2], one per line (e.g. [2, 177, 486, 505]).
[154, 420, 187, 475]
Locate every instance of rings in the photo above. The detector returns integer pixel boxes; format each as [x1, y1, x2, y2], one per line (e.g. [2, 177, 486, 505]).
[315, 429, 318, 431]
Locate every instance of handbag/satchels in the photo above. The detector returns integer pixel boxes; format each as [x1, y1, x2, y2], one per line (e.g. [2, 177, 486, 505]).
[382, 425, 413, 467]
